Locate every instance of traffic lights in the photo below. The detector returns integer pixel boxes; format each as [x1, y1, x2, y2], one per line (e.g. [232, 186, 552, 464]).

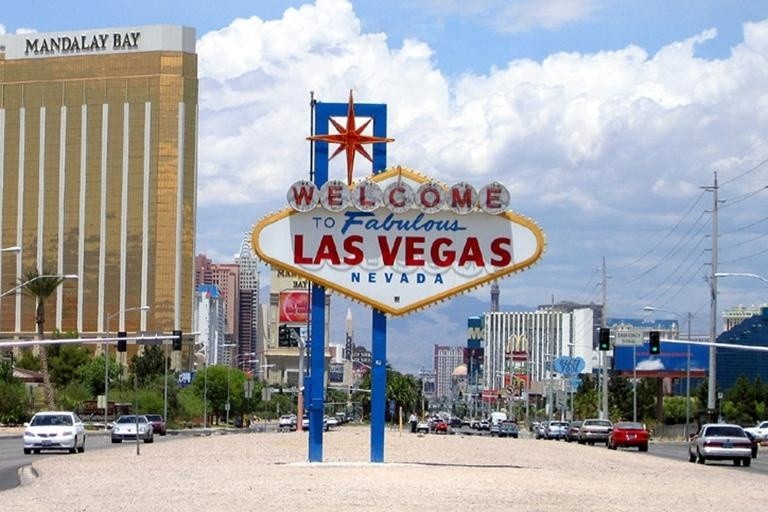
[649, 329, 660, 355]
[598, 329, 610, 351]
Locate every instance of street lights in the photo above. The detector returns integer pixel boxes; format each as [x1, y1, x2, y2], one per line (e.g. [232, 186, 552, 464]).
[417, 352, 574, 422]
[103, 304, 150, 432]
[163, 331, 201, 426]
[712, 270, 767, 284]
[0, 244, 21, 254]
[202, 342, 236, 429]
[0, 273, 79, 301]
[642, 307, 690, 440]
[349, 359, 371, 370]
[595, 326, 637, 423]
[225, 351, 276, 428]
[569, 343, 601, 419]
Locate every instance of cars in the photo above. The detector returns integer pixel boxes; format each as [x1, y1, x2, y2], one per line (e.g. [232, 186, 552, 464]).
[111, 414, 154, 443]
[415, 413, 583, 441]
[687, 423, 752, 467]
[276, 402, 353, 441]
[22, 410, 87, 455]
[744, 419, 768, 443]
[606, 421, 650, 454]
[745, 430, 757, 459]
[142, 413, 166, 436]
[576, 418, 612, 446]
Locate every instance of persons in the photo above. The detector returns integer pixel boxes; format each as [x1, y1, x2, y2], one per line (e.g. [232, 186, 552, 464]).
[408, 412, 419, 432]
